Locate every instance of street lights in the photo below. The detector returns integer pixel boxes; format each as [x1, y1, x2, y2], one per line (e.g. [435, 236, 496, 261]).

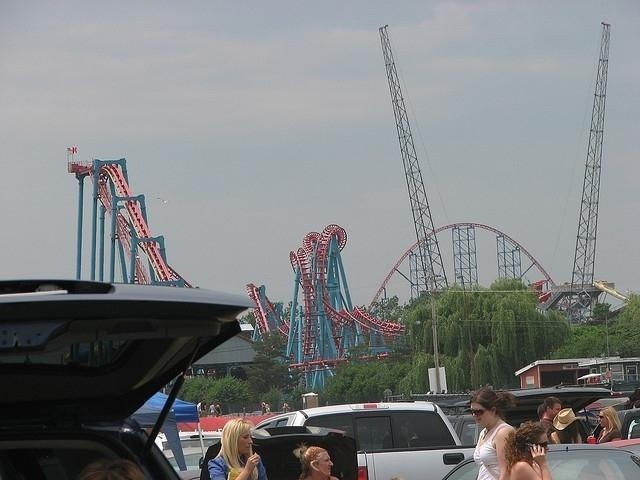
[418, 273, 446, 395]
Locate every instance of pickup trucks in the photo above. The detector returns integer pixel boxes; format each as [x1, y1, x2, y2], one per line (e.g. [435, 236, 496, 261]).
[210, 395, 481, 479]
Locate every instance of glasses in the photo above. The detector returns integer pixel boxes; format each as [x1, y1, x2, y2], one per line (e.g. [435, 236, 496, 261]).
[531, 441, 548, 448]
[469, 407, 488, 417]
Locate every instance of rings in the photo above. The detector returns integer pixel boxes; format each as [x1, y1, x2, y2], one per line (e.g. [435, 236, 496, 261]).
[534, 447, 537, 450]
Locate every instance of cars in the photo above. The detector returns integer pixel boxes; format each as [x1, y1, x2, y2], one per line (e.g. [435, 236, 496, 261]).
[0, 275, 262, 480]
[437, 440, 640, 480]
[444, 386, 640, 447]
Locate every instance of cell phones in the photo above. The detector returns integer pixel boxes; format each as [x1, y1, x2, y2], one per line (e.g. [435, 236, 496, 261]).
[525, 443, 537, 453]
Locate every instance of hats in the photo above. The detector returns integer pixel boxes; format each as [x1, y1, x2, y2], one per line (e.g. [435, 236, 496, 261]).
[552, 407, 577, 431]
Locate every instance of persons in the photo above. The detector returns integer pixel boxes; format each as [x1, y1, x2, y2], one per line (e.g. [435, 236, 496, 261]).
[467, 388, 518, 480]
[504, 419, 553, 480]
[293, 440, 341, 480]
[77, 457, 148, 480]
[534, 366, 628, 444]
[196, 398, 292, 416]
[204, 417, 270, 479]
